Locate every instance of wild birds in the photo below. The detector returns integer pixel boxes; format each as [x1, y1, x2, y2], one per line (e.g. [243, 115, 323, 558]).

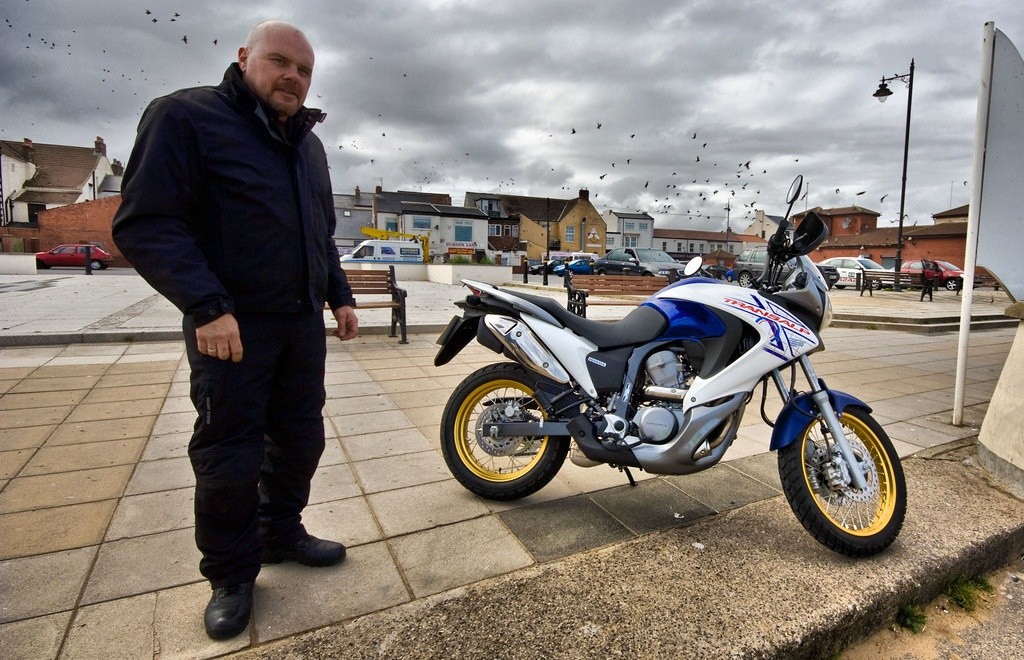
[0, 9, 218, 133]
[315, 55, 969, 226]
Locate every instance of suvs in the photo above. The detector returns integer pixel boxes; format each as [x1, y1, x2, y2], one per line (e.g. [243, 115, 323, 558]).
[733, 242, 841, 291]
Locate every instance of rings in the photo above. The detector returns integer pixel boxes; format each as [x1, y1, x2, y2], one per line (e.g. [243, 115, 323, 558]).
[208, 348, 216, 353]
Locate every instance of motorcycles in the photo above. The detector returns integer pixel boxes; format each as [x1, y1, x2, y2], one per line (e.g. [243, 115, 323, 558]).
[432, 175, 908, 558]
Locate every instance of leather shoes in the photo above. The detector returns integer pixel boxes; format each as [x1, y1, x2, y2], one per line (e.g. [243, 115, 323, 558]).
[263, 532, 345, 567]
[204, 583, 254, 639]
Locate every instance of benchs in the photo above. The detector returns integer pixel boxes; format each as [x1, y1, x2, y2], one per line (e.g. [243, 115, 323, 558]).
[324, 265, 409, 345]
[858, 268, 934, 302]
[563, 269, 676, 319]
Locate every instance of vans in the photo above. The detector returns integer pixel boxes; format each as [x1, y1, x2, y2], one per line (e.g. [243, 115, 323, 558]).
[338, 240, 424, 264]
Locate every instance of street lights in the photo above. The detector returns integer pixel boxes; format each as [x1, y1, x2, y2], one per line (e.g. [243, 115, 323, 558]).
[873, 56, 916, 293]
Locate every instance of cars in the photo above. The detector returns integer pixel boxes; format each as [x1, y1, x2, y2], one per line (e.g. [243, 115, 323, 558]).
[595, 246, 700, 287]
[816, 256, 900, 292]
[528, 260, 567, 276]
[701, 263, 732, 281]
[35, 243, 115, 271]
[896, 258, 966, 291]
[552, 258, 596, 278]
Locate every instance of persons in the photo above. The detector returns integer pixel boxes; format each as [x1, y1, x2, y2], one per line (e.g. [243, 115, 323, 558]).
[110, 20, 358, 640]
